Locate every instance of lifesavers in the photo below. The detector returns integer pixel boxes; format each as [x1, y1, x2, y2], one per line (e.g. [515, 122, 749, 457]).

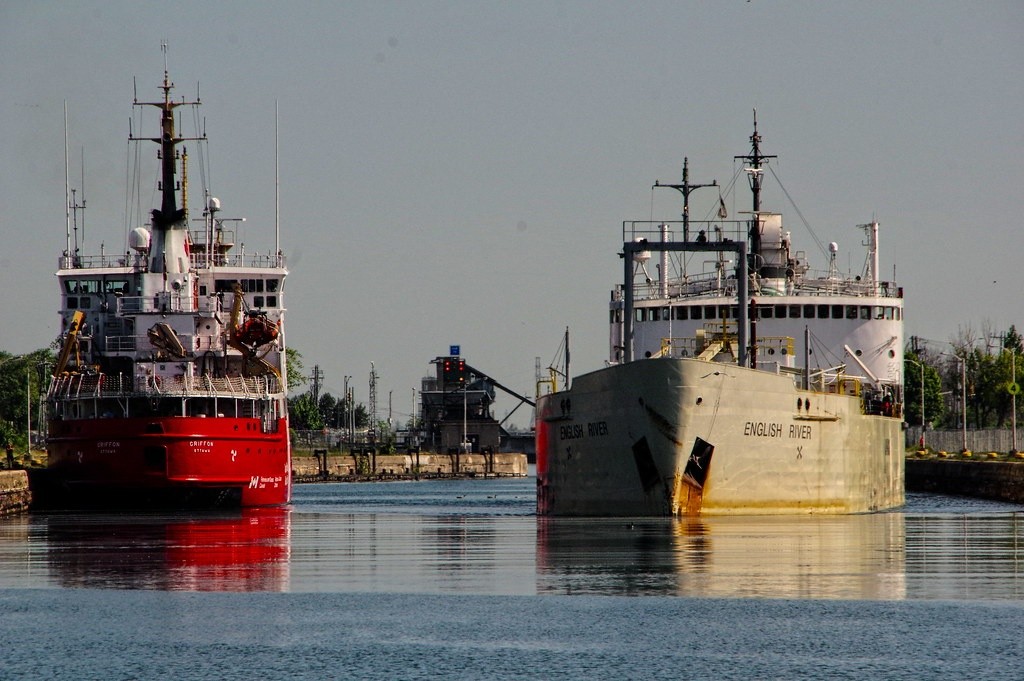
[148, 376, 161, 390]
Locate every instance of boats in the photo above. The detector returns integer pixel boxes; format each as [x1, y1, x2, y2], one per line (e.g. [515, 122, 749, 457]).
[533, 107, 905, 516]
[43, 38, 292, 515]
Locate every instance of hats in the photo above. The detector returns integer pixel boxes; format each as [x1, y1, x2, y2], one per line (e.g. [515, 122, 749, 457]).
[887, 392, 891, 396]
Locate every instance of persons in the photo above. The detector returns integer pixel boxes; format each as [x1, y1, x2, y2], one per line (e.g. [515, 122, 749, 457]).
[920, 436, 924, 450]
[883, 392, 891, 416]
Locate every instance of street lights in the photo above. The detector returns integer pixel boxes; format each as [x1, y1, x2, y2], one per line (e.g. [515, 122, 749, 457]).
[904, 359, 925, 453]
[939, 351, 966, 452]
[987, 344, 1016, 455]
